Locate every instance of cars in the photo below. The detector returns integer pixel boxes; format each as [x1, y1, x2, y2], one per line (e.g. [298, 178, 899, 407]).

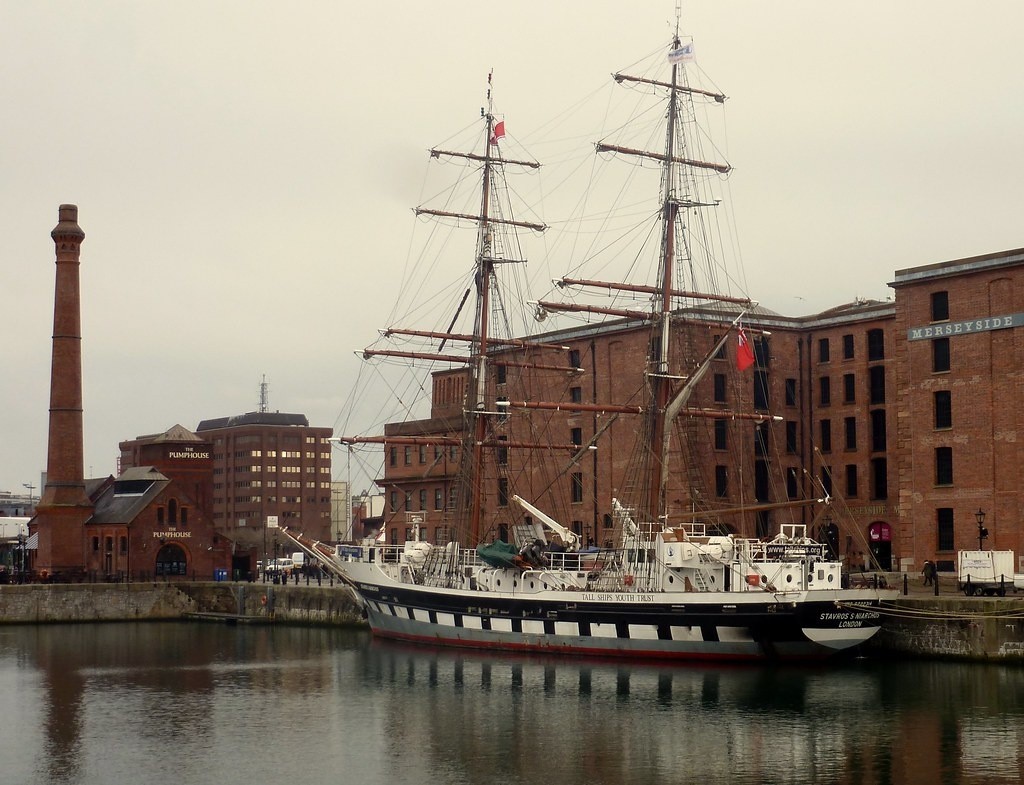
[266, 558, 294, 575]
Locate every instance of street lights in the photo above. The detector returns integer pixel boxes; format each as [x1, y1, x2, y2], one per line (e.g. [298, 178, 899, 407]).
[336, 529, 342, 544]
[15, 523, 24, 576]
[0, 522, 9, 540]
[975, 507, 985, 550]
[272, 531, 278, 576]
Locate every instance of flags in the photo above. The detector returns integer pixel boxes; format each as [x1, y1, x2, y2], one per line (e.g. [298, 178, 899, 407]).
[737, 327, 755, 373]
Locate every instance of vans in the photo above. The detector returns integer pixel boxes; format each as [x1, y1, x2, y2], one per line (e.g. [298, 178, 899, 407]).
[291, 552, 304, 569]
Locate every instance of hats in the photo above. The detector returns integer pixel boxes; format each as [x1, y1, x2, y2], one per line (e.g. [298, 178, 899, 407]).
[924, 560, 928, 562]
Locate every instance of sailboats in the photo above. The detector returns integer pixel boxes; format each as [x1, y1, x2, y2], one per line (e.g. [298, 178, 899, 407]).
[268, 2, 902, 665]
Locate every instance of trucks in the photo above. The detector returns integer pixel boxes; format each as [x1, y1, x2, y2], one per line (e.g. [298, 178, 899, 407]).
[956, 548, 1015, 597]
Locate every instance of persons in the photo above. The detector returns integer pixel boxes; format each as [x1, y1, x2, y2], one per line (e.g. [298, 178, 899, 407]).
[921, 559, 937, 586]
[761, 535, 772, 543]
[519, 538, 548, 569]
